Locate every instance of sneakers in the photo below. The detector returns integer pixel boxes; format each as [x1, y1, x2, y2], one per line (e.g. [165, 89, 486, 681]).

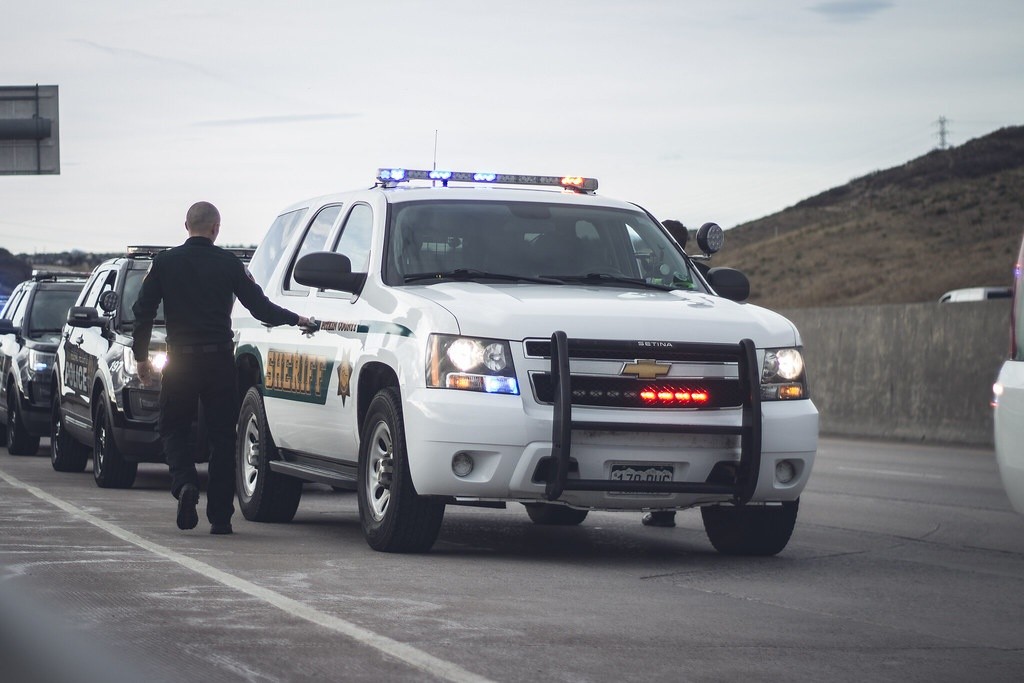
[176, 483, 200, 530]
[208, 517, 233, 535]
[641, 512, 674, 526]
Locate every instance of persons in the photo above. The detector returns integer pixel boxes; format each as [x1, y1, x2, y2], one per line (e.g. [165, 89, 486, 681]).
[638, 219, 715, 527]
[131, 201, 309, 534]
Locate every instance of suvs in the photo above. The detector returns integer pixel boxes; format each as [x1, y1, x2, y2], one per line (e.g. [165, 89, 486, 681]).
[220, 166, 819, 559]
[0, 263, 92, 458]
[50, 242, 249, 489]
